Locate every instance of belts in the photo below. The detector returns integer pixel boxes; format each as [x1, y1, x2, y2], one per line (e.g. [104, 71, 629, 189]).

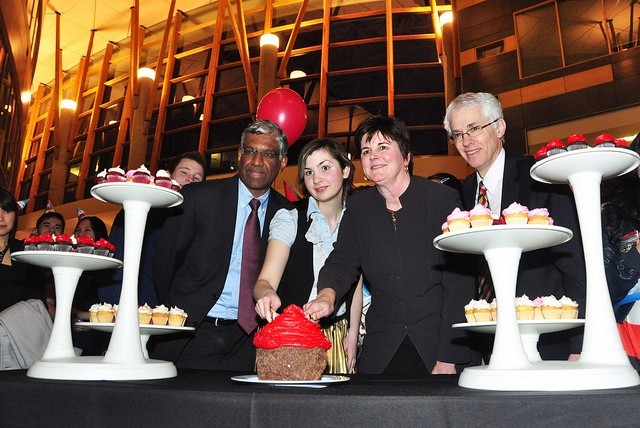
[204, 315, 237, 327]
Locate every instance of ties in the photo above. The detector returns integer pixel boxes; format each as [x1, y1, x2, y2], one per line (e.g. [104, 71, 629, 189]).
[476, 181, 496, 304]
[236, 198, 264, 335]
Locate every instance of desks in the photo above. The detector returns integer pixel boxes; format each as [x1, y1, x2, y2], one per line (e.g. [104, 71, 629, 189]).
[1, 369, 637, 428]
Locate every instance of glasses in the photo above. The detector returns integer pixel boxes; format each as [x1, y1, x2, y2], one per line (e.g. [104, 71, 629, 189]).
[242, 146, 280, 159]
[448, 118, 499, 144]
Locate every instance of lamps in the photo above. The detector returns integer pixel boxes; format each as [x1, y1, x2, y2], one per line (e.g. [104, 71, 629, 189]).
[289, 70, 307, 79]
[439, 11, 453, 26]
[259, 34, 279, 48]
[181, 95, 196, 107]
[59, 98, 77, 111]
[138, 68, 156, 81]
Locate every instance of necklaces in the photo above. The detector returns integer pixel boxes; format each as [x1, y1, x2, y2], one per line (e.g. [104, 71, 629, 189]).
[0, 245, 10, 263]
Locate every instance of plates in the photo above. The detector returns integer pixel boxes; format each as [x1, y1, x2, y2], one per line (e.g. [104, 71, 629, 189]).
[231, 375, 351, 389]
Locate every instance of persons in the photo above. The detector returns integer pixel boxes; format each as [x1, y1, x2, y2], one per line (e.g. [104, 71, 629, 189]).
[0, 187, 48, 311]
[145, 120, 295, 373]
[302, 114, 483, 385]
[443, 91, 586, 365]
[601, 133, 640, 378]
[71, 215, 109, 245]
[252, 137, 365, 374]
[426, 172, 464, 202]
[96, 150, 207, 307]
[33, 211, 65, 237]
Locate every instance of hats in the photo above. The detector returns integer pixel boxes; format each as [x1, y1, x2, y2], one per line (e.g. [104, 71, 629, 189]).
[17, 198, 30, 211]
[77, 207, 87, 220]
[44, 199, 55, 213]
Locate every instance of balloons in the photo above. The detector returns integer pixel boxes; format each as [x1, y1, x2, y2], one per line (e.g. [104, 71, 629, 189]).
[255, 87, 308, 148]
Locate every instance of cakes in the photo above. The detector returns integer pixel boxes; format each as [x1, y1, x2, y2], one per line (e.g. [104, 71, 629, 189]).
[97, 302, 113, 323]
[138, 302, 152, 324]
[174, 306, 189, 326]
[254, 304, 333, 380]
[132, 164, 151, 185]
[70, 235, 78, 252]
[55, 234, 72, 252]
[97, 168, 108, 183]
[37, 234, 55, 251]
[516, 294, 534, 320]
[470, 203, 494, 227]
[567, 134, 589, 152]
[559, 296, 576, 320]
[88, 302, 102, 322]
[474, 299, 491, 321]
[464, 298, 476, 322]
[172, 179, 181, 191]
[546, 138, 567, 156]
[593, 133, 616, 149]
[491, 297, 498, 321]
[126, 170, 136, 182]
[78, 236, 94, 254]
[615, 139, 630, 147]
[152, 304, 169, 325]
[542, 295, 562, 321]
[169, 306, 184, 326]
[441, 221, 448, 233]
[528, 208, 550, 225]
[113, 303, 119, 323]
[154, 169, 172, 190]
[534, 296, 543, 320]
[109, 242, 115, 257]
[108, 165, 126, 181]
[94, 237, 109, 257]
[502, 201, 529, 225]
[534, 145, 547, 161]
[149, 175, 156, 185]
[447, 208, 470, 232]
[24, 235, 37, 251]
